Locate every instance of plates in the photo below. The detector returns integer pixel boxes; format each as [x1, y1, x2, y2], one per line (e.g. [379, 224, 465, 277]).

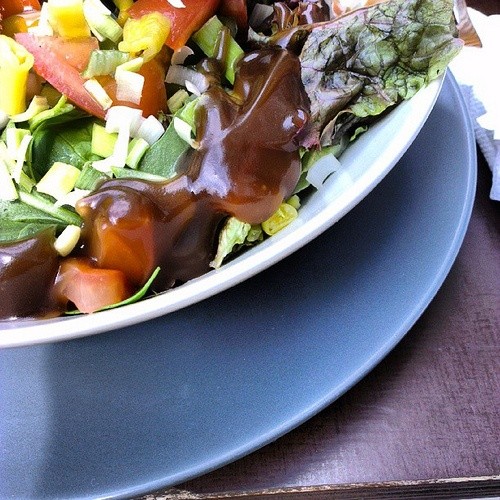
[0, 0, 448, 346]
[0, 60, 475, 500]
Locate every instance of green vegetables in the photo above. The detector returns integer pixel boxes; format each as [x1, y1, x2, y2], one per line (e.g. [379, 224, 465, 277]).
[0, 0, 464, 316]
